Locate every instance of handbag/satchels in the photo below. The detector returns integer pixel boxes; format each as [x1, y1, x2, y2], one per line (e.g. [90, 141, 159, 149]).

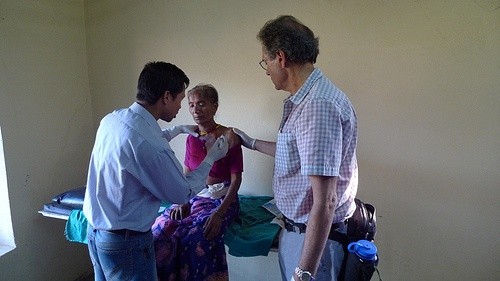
[328, 198, 378, 254]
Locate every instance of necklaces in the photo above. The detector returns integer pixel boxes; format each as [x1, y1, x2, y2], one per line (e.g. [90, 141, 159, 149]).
[198, 123, 220, 136]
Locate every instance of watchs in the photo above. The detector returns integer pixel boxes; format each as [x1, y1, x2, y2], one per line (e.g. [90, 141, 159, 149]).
[294, 267, 315, 281]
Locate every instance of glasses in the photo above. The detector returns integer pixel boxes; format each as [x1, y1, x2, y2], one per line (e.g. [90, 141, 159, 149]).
[259, 48, 281, 71]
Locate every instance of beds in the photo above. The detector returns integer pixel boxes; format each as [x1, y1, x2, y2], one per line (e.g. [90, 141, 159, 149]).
[37, 187, 278, 257]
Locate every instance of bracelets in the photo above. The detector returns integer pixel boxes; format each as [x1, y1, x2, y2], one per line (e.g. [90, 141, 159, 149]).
[214, 211, 225, 218]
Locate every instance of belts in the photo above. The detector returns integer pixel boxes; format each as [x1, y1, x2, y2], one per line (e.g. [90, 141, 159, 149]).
[281, 214, 344, 234]
[105, 228, 151, 237]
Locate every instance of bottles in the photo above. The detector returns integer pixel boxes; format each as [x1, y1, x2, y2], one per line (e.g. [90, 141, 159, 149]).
[348, 240, 378, 264]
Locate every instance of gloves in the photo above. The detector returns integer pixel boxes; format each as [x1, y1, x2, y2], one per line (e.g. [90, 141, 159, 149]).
[202, 134, 229, 167]
[170, 124, 200, 137]
[228, 126, 257, 151]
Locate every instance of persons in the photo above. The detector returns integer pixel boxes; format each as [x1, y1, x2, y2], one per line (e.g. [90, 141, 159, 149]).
[152, 84, 243, 281]
[228, 14, 359, 281]
[84, 60, 228, 281]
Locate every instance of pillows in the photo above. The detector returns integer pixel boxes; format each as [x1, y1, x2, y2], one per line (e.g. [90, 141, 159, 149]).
[52, 189, 84, 210]
